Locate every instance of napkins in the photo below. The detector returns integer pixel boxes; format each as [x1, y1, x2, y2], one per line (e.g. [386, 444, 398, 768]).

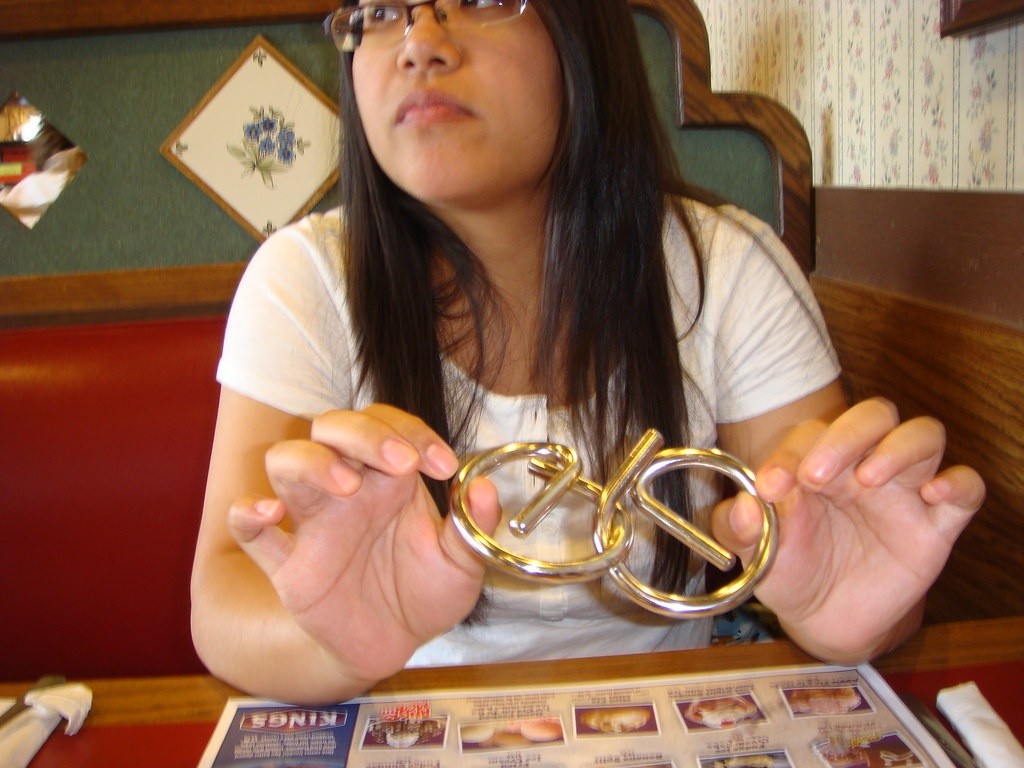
[0, 683, 92, 768]
[934, 680, 1024, 768]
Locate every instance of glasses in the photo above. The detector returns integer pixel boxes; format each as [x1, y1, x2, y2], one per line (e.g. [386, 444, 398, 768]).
[322, 0, 527, 51]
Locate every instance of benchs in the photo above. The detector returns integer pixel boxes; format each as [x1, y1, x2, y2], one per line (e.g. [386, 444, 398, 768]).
[0, 317, 231, 683]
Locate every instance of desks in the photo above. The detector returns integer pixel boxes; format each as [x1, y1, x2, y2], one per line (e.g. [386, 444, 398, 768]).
[0, 608, 1024, 768]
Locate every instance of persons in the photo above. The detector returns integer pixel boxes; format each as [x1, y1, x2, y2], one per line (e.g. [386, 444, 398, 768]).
[191, 0, 988, 709]
